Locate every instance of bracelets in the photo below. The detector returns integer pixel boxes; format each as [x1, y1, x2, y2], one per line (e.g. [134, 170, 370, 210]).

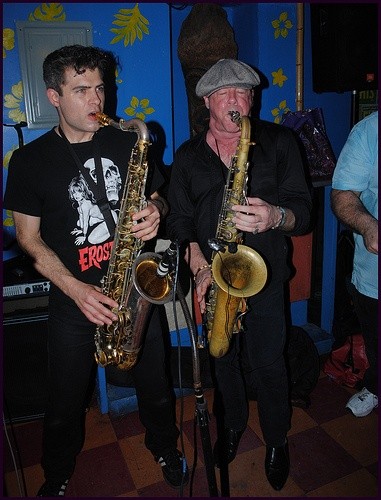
[271, 205, 286, 230]
[151, 199, 164, 213]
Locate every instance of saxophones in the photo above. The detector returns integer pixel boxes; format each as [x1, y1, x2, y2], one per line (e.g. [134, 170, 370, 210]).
[89, 110, 175, 369]
[201, 111, 269, 358]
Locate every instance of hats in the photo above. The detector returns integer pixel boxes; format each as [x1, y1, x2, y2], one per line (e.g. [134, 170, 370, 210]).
[195, 58, 261, 97]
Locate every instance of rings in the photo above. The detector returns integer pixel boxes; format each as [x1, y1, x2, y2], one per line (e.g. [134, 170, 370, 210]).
[254, 222, 260, 235]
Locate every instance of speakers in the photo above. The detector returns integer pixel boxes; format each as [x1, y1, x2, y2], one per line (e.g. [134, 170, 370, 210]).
[2, 314, 89, 423]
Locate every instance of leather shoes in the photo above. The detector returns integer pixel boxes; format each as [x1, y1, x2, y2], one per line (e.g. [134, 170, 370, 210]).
[213, 423, 247, 469]
[264, 435, 291, 491]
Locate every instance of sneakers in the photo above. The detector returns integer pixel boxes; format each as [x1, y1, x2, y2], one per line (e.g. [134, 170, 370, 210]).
[36, 479, 70, 497]
[155, 448, 190, 489]
[345, 386, 379, 417]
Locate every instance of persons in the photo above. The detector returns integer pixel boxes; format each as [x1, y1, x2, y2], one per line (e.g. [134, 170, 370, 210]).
[2, 43, 185, 497]
[168, 58, 314, 496]
[331, 111, 379, 419]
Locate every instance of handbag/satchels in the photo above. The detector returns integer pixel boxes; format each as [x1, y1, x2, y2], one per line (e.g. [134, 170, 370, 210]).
[321, 335, 370, 395]
[278, 107, 338, 188]
[241, 325, 321, 409]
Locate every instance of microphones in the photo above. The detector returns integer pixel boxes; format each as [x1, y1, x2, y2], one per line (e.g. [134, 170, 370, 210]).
[156, 242, 177, 278]
